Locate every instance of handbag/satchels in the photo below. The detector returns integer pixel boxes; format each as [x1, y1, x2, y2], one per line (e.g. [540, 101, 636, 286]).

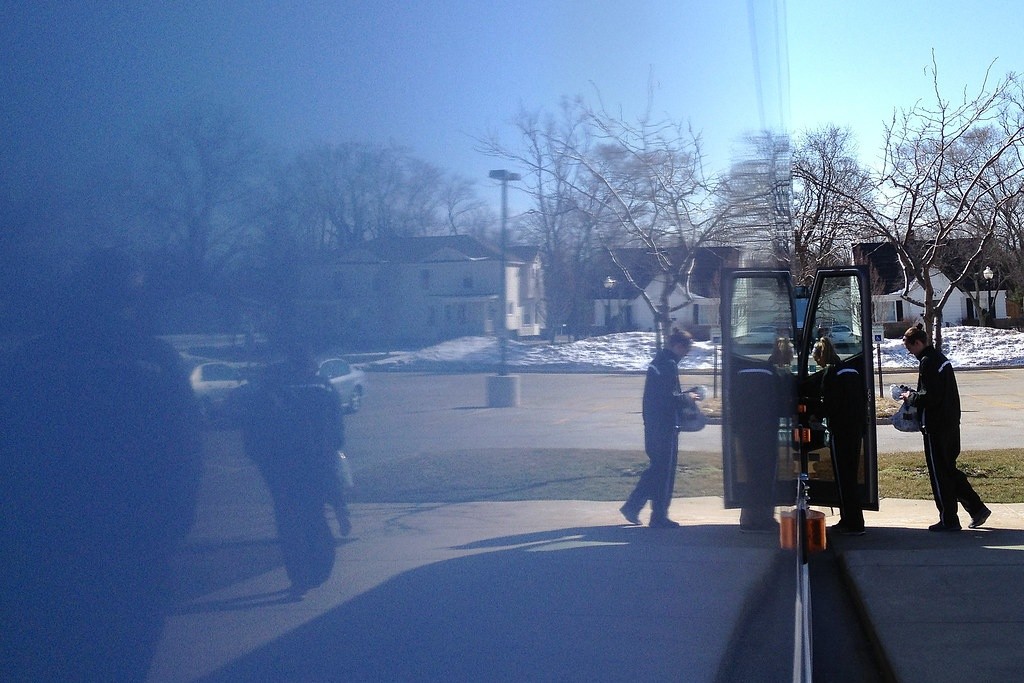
[680, 393, 705, 431]
[331, 450, 354, 489]
[889, 392, 921, 432]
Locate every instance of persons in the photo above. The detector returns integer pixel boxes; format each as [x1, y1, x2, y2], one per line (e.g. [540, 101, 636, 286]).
[899, 323, 991, 533]
[732, 337, 794, 532]
[812, 337, 866, 535]
[620, 326, 701, 528]
[0, 243, 351, 683]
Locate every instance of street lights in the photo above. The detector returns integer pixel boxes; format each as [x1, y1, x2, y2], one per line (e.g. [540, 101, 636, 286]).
[488, 168, 522, 375]
[983, 266, 994, 327]
[602, 276, 616, 333]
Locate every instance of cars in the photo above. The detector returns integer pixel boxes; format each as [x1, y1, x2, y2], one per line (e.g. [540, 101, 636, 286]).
[829, 324, 856, 346]
[187, 360, 250, 424]
[314, 355, 368, 413]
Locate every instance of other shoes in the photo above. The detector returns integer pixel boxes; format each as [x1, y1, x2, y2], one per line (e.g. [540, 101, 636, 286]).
[738, 517, 781, 533]
[619, 505, 642, 525]
[649, 514, 679, 528]
[969, 506, 991, 528]
[840, 525, 866, 535]
[831, 522, 844, 531]
[929, 521, 962, 531]
